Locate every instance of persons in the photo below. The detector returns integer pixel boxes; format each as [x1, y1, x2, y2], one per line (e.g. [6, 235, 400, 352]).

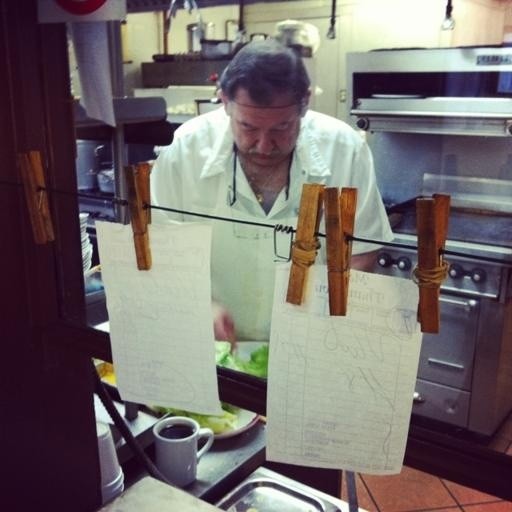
[150, 40, 395, 341]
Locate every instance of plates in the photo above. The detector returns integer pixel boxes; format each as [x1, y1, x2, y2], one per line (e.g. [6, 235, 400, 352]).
[79, 212, 93, 273]
[237, 340, 269, 363]
[213, 406, 259, 440]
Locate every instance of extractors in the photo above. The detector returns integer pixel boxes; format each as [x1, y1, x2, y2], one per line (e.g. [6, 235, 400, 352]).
[344, 46, 511, 138]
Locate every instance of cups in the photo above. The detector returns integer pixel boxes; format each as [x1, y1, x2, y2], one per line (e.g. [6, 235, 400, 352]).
[153, 415, 215, 489]
[95, 422, 125, 497]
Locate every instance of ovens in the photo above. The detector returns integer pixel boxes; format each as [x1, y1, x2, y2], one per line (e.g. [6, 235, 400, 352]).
[375, 281, 512, 437]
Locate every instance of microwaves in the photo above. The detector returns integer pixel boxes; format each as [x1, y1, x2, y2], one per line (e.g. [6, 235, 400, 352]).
[374, 193, 511, 298]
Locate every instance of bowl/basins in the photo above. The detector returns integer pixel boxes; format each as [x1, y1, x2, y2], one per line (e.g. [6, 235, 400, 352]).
[199, 38, 233, 60]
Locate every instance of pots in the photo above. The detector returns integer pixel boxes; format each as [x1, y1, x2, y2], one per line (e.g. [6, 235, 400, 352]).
[74, 139, 118, 194]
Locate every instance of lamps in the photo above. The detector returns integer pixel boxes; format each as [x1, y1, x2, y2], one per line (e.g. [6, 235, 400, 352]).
[440, 0, 456, 30]
[326, 0, 337, 38]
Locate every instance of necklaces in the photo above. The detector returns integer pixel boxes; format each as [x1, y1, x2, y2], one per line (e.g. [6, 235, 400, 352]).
[239, 163, 274, 207]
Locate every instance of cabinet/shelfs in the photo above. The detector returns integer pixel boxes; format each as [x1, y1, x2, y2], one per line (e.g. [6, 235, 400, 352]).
[65, 0, 166, 333]
